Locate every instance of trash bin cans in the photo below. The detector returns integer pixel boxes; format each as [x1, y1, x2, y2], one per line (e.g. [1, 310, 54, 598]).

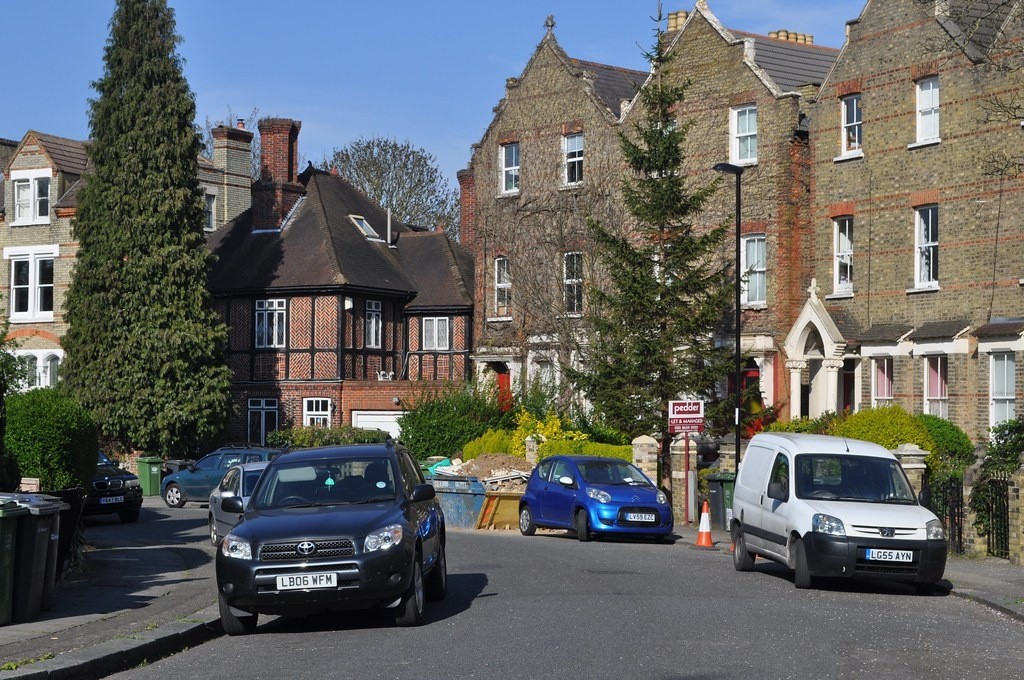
[707, 474, 725, 530]
[166, 458, 195, 477]
[0, 499, 29, 625]
[0, 495, 60, 622]
[136, 457, 161, 496]
[721, 473, 736, 532]
[43, 502, 70, 597]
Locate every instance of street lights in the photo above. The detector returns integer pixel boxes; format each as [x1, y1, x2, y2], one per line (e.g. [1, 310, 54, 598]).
[711, 162, 744, 476]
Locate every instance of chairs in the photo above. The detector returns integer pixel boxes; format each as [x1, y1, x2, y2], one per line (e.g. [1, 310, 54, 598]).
[365, 463, 389, 487]
[296, 481, 319, 496]
[845, 466, 866, 491]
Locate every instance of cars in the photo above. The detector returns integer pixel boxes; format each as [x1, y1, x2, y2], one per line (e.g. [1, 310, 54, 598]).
[517, 453, 674, 542]
[209, 461, 275, 549]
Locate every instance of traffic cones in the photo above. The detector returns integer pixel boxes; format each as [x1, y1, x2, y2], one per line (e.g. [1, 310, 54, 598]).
[689, 501, 721, 552]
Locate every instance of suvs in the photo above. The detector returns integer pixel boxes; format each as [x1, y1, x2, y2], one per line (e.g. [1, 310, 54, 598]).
[729, 430, 949, 589]
[159, 446, 282, 507]
[80, 450, 144, 523]
[213, 440, 446, 635]
